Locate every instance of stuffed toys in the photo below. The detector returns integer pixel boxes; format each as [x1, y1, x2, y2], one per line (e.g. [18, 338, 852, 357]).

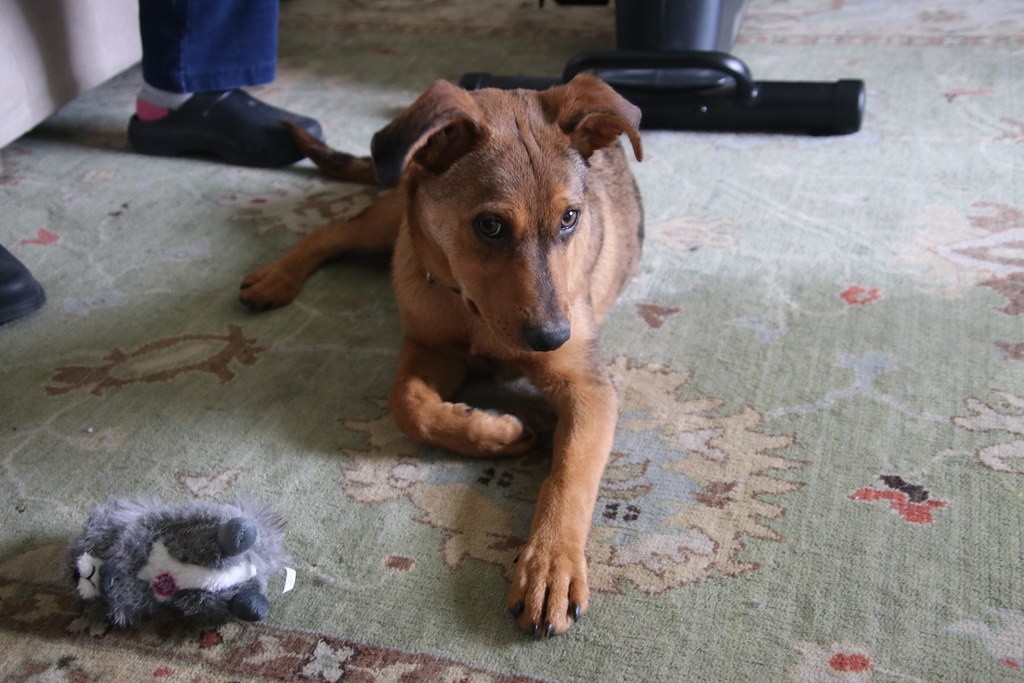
[66, 495, 291, 636]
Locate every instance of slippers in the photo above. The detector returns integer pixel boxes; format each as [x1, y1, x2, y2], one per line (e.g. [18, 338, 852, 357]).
[125, 89, 319, 166]
[0, 249, 46, 326]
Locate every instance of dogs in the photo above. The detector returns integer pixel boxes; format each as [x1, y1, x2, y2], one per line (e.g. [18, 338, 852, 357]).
[234, 70, 645, 642]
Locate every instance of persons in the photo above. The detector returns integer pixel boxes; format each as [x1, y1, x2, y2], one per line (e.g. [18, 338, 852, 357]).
[0, 0, 325, 326]
